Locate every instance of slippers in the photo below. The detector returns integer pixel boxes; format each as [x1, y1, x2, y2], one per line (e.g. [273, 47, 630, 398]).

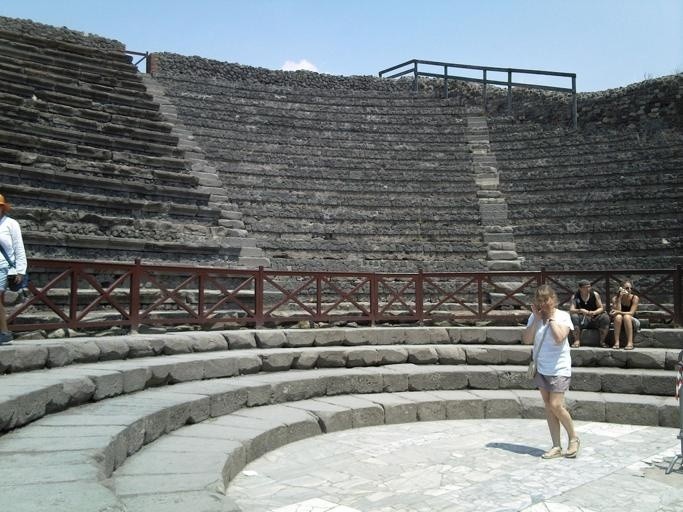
[600, 340, 634, 350]
[571, 342, 581, 347]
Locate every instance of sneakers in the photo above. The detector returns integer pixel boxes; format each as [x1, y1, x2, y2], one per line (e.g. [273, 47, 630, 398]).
[0, 331, 15, 345]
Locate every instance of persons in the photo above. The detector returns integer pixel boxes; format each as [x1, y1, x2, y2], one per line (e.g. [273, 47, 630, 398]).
[519, 284, 584, 462]
[607, 279, 642, 351]
[566, 278, 611, 346]
[0, 192, 29, 349]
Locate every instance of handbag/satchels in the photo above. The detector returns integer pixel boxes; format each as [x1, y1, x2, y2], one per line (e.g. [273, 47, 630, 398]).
[526, 360, 538, 381]
[7, 272, 30, 292]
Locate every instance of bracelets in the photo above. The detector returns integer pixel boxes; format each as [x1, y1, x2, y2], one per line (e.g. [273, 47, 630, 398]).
[546, 318, 554, 323]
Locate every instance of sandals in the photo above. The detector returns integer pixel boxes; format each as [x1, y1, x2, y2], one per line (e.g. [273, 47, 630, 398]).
[542, 446, 563, 459]
[566, 435, 580, 458]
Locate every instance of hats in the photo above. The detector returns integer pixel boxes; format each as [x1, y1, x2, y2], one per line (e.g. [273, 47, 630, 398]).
[0, 195, 12, 212]
[578, 280, 591, 288]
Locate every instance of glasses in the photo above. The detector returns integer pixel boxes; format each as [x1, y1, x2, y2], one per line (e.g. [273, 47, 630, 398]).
[624, 287, 633, 290]
